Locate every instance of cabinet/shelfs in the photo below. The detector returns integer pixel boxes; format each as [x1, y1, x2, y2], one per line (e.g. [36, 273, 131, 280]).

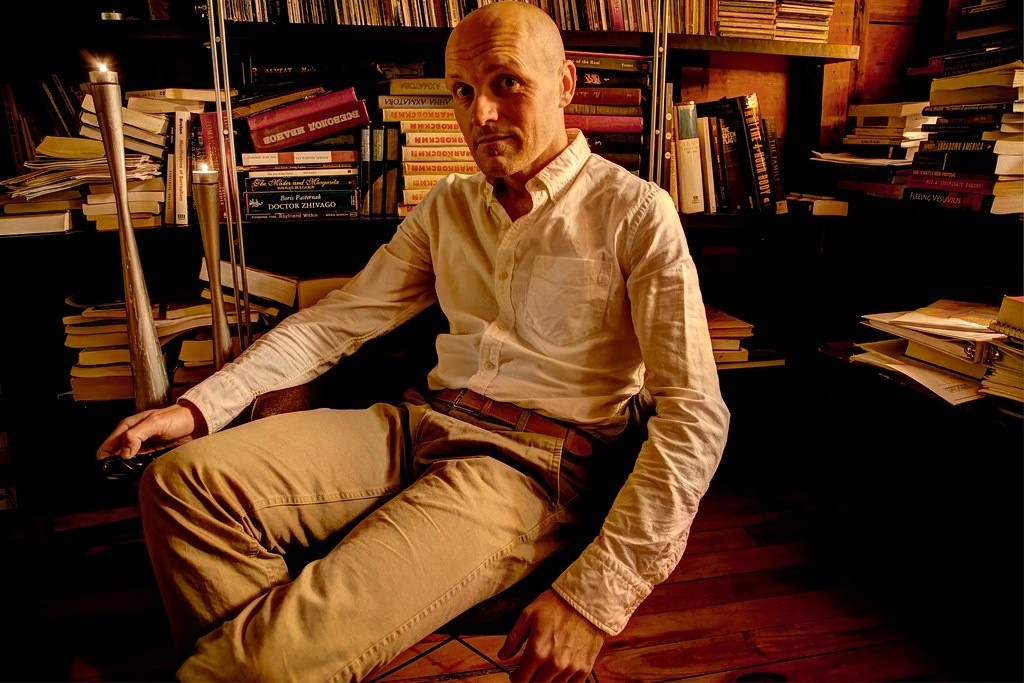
[0, 0, 1024, 683]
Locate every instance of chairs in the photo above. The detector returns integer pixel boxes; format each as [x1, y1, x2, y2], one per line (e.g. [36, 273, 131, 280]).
[251, 306, 656, 683]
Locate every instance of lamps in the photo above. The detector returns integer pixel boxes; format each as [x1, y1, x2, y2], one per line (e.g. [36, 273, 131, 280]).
[89, 71, 119, 85]
[192, 170, 219, 185]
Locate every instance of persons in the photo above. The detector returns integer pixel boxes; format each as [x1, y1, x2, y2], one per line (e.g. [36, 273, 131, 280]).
[94, 0, 731, 682]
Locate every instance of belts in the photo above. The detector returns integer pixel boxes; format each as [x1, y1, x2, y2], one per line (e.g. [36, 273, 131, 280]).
[436, 386, 607, 458]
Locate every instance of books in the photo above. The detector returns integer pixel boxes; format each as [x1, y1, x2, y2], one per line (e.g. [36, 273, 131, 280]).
[0, 0, 1024, 473]
